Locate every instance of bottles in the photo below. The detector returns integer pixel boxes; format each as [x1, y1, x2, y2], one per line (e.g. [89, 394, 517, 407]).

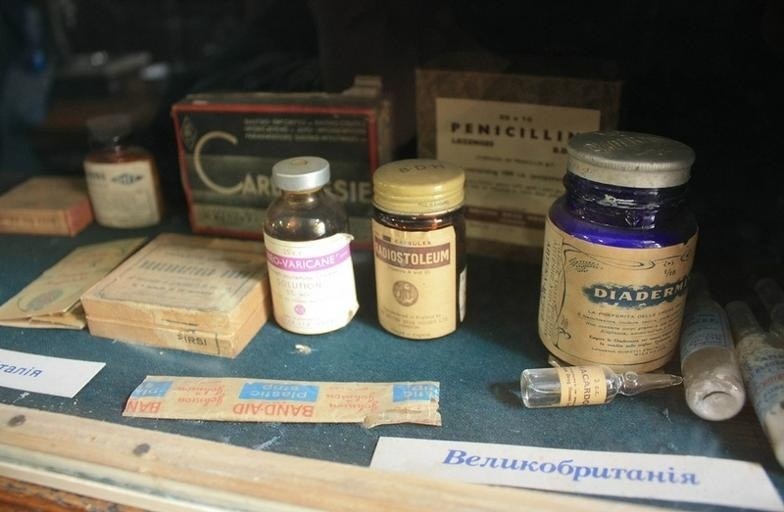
[682, 273, 783, 465]
[262, 155, 360, 336]
[521, 363, 686, 409]
[81, 129, 162, 229]
[369, 154, 473, 339]
[539, 128, 703, 372]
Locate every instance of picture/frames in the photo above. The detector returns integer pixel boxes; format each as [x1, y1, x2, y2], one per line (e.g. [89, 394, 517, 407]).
[414, 68, 618, 266]
